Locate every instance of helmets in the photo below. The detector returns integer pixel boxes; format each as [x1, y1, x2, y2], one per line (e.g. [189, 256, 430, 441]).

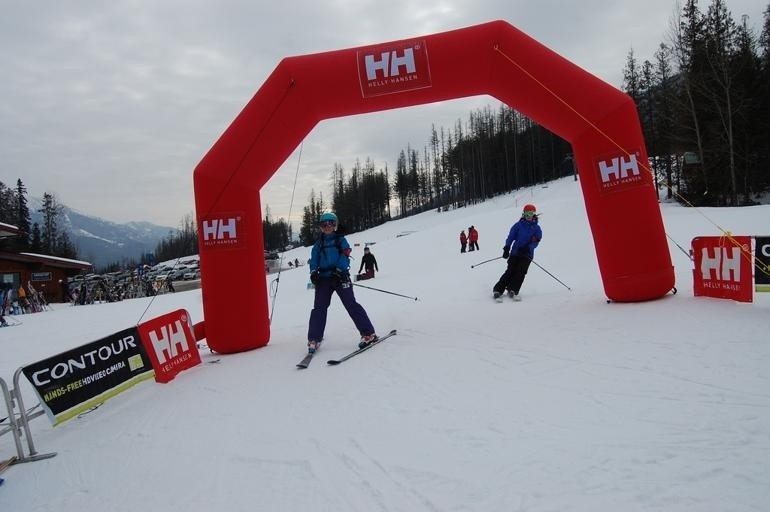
[320, 213, 338, 222]
[523, 204, 536, 213]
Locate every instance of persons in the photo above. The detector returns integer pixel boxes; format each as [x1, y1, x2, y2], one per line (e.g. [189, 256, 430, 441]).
[469, 225, 479, 250]
[356, 247, 380, 280]
[460, 230, 468, 254]
[466, 227, 473, 252]
[295, 258, 298, 267]
[305, 212, 379, 352]
[491, 204, 542, 298]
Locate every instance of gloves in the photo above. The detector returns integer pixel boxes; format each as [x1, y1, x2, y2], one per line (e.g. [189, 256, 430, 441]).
[330, 269, 342, 284]
[519, 244, 529, 254]
[503, 246, 510, 258]
[310, 270, 320, 285]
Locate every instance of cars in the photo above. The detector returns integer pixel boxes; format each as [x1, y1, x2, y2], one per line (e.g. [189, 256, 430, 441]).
[284, 244, 294, 251]
[57, 259, 200, 301]
[263, 248, 279, 260]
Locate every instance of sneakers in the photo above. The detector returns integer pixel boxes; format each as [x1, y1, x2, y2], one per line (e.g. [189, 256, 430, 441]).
[493, 287, 500, 298]
[509, 289, 516, 297]
[308, 340, 321, 349]
[358, 333, 376, 348]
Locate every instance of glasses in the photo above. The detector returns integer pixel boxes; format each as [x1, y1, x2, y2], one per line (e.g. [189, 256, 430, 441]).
[523, 211, 535, 217]
[319, 220, 337, 228]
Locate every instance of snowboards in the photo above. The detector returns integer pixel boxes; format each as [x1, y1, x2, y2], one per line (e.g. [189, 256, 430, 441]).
[355, 273, 372, 280]
[0, 281, 53, 316]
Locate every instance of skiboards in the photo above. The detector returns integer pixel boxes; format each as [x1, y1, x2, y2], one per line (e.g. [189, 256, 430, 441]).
[294, 329, 395, 368]
[75, 268, 174, 304]
[493, 288, 523, 305]
